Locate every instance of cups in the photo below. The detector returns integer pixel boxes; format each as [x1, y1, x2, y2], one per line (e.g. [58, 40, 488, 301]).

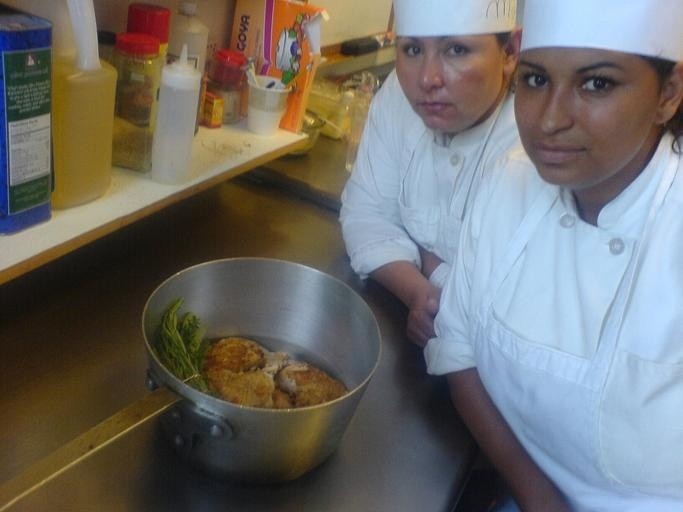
[247, 74, 291, 134]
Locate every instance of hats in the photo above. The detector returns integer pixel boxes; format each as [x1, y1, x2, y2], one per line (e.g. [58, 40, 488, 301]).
[520, 0, 683, 63]
[392, 0, 518, 37]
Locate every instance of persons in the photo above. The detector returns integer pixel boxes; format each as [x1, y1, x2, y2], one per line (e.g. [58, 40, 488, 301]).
[423, 45, 683, 512]
[338, 27, 521, 348]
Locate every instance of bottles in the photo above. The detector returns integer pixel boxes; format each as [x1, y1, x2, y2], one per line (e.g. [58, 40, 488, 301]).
[110, 0, 244, 182]
[345, 84, 370, 170]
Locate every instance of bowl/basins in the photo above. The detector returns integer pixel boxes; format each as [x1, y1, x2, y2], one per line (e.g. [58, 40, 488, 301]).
[287, 105, 325, 157]
